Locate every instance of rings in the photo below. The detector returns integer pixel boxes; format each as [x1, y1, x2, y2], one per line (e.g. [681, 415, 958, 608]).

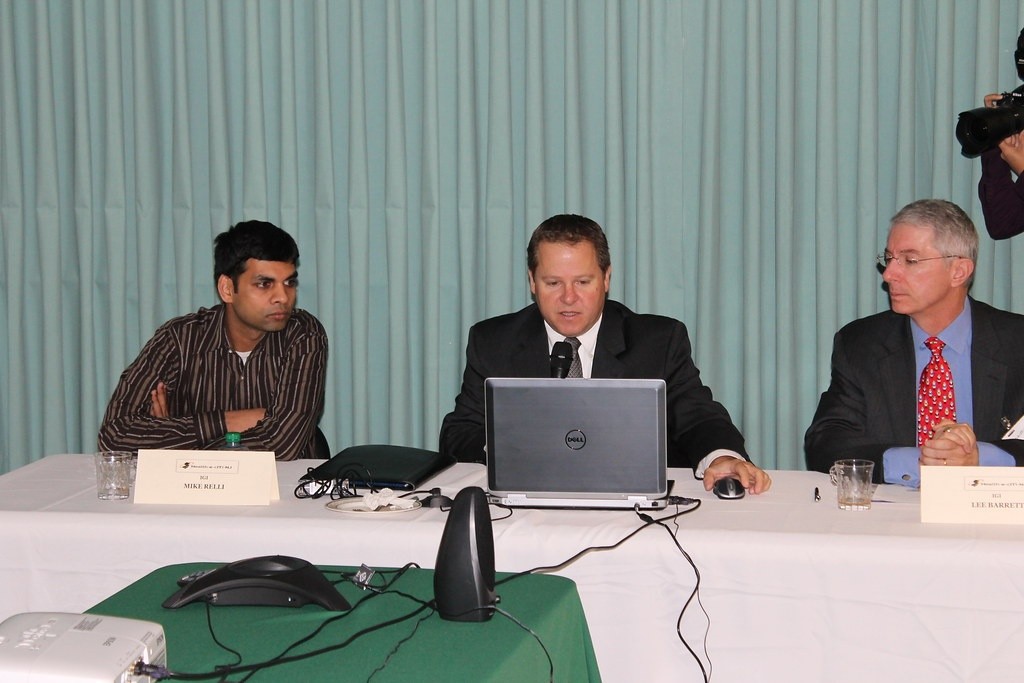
[943, 459, 946, 466]
[943, 426, 952, 433]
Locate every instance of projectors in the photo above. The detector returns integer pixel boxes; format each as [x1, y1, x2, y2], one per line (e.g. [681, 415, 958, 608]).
[0, 612, 167, 683]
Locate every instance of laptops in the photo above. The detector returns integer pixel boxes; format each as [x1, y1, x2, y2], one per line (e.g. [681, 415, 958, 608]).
[484, 377, 675, 510]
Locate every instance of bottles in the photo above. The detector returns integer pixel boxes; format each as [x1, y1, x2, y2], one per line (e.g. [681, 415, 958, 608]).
[218, 432, 249, 451]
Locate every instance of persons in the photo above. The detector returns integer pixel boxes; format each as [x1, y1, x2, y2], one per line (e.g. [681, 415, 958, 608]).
[97, 218, 330, 461]
[804, 198, 1024, 491]
[438, 214, 772, 496]
[978, 91, 1024, 241]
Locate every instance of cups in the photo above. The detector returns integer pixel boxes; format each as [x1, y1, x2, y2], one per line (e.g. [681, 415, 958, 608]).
[94, 451, 132, 500]
[830, 459, 875, 510]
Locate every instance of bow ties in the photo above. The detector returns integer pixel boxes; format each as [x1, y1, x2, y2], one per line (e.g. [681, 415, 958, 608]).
[918, 336, 957, 446]
[563, 337, 583, 378]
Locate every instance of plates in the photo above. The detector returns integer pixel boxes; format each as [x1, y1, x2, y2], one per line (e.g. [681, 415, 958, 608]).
[326, 497, 422, 514]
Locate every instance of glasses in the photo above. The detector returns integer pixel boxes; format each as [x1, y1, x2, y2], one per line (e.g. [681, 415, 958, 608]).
[877, 252, 961, 268]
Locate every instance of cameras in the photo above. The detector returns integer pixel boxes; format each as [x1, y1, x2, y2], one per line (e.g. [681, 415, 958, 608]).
[955, 83, 1024, 159]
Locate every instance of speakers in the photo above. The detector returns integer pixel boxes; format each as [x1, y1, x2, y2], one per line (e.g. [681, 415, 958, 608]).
[435, 486, 497, 621]
[162, 555, 352, 613]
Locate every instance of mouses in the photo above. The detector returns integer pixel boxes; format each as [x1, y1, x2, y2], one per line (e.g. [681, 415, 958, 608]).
[712, 477, 745, 500]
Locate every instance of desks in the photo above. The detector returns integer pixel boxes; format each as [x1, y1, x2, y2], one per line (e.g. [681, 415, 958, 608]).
[81, 561, 604, 683]
[0, 453, 1024, 683]
